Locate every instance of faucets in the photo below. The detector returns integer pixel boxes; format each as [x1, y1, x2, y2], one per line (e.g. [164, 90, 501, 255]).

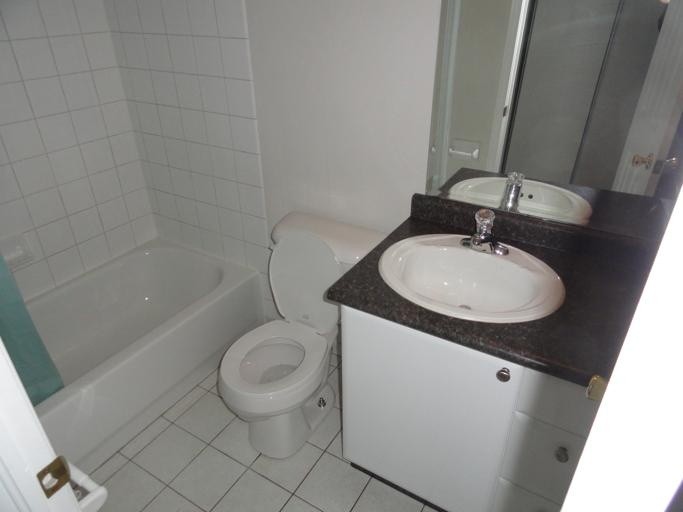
[498, 171, 526, 214]
[461, 208, 509, 256]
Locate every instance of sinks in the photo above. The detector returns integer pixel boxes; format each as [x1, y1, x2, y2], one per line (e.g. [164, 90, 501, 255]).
[378, 234, 566, 325]
[446, 176, 593, 227]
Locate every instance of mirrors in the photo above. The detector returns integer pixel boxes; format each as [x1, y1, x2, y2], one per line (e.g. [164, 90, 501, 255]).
[424, 1, 683, 247]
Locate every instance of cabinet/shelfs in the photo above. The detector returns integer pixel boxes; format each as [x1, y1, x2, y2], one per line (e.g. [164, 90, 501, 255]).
[339, 307, 527, 512]
[487, 367, 606, 512]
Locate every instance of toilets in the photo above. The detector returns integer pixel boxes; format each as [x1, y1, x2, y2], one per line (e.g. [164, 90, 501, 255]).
[216, 210, 391, 460]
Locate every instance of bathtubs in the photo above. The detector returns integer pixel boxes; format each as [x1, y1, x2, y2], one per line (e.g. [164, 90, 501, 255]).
[24, 233, 261, 477]
